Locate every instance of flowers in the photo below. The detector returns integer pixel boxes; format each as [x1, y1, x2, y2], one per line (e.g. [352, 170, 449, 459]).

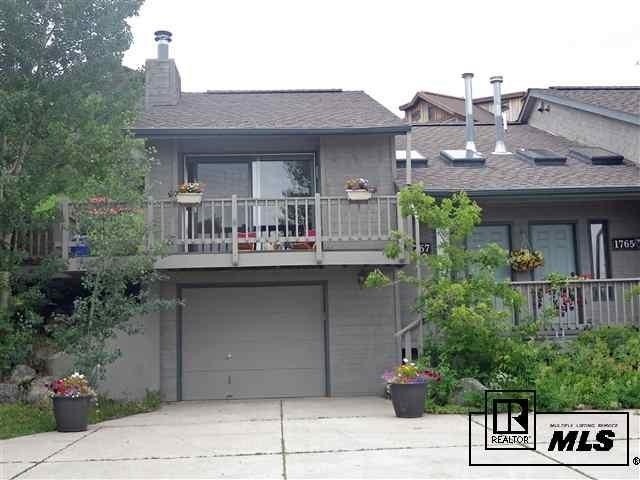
[179, 180, 206, 193]
[382, 357, 441, 385]
[344, 175, 369, 189]
[47, 371, 100, 399]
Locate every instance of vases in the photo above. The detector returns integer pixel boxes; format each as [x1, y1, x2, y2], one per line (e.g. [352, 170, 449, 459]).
[344, 189, 372, 201]
[175, 192, 203, 203]
[52, 394, 92, 432]
[390, 383, 428, 418]
